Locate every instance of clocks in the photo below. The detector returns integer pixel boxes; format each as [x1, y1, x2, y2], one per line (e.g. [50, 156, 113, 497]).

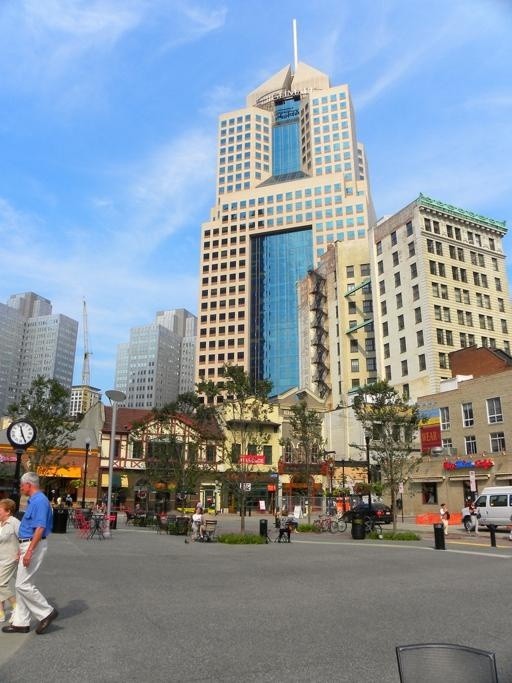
[6, 418, 38, 452]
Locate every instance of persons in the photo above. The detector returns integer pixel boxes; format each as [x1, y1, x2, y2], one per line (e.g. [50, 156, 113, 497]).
[0, 498, 24, 621]
[191, 506, 202, 539]
[276, 509, 293, 542]
[274, 503, 301, 534]
[2, 470, 59, 634]
[199, 508, 216, 542]
[65, 493, 73, 506]
[466, 499, 479, 534]
[57, 494, 63, 505]
[439, 502, 449, 535]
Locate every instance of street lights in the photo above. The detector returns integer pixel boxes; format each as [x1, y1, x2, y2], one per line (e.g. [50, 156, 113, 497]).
[81, 436, 91, 509]
[340, 455, 346, 513]
[364, 429, 372, 507]
[329, 462, 335, 506]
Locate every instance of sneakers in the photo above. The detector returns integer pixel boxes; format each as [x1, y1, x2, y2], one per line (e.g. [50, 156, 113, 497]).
[0, 614, 5, 622]
[8, 609, 17, 624]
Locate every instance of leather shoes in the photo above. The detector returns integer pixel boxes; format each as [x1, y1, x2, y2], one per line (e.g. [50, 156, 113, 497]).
[35, 608, 58, 634]
[2, 624, 30, 633]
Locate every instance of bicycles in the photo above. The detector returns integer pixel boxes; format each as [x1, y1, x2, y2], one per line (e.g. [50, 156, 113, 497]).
[311, 514, 347, 533]
[363, 518, 382, 535]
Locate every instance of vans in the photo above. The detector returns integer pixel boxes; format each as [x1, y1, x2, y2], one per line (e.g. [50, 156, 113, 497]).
[461, 486, 512, 533]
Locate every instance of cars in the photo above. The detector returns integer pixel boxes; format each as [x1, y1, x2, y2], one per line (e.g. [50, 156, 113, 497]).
[342, 502, 395, 525]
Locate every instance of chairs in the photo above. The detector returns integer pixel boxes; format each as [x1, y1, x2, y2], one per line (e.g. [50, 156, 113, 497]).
[65, 506, 218, 545]
[395, 642, 499, 683]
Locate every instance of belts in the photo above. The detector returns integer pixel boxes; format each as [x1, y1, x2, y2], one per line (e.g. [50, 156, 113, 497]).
[19, 536, 46, 543]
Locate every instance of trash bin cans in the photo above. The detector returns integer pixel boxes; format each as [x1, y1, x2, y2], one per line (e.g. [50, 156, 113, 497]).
[110, 512, 117, 530]
[176, 516, 189, 535]
[260, 518, 268, 537]
[352, 517, 364, 539]
[53, 507, 68, 534]
[434, 523, 445, 550]
